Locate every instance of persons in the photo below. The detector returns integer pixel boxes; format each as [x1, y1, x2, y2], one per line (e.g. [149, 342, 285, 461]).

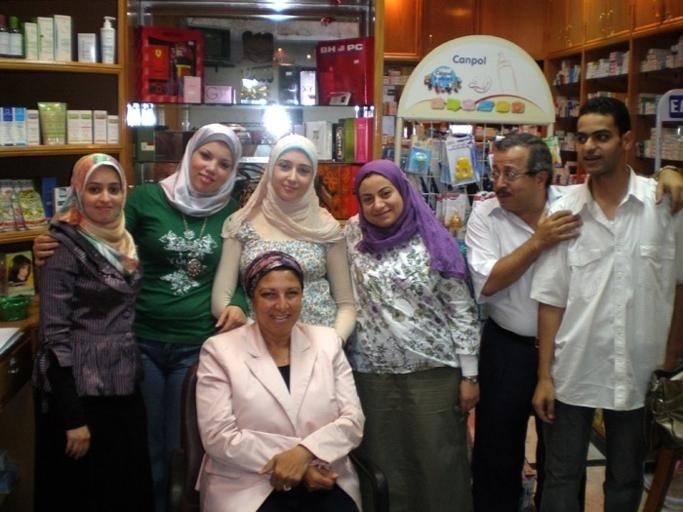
[527, 95, 682, 509]
[187, 248, 365, 510]
[28, 152, 146, 511]
[208, 135, 357, 353]
[462, 130, 682, 510]
[3, 255, 31, 284]
[28, 121, 254, 512]
[343, 154, 485, 511]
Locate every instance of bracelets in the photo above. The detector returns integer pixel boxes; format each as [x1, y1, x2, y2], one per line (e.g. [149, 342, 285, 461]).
[651, 163, 679, 180]
[462, 376, 479, 384]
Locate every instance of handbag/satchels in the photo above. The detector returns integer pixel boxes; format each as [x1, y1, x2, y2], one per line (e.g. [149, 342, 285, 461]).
[643, 361, 683, 454]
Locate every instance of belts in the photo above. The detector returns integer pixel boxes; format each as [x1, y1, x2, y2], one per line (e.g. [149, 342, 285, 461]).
[485, 314, 542, 351]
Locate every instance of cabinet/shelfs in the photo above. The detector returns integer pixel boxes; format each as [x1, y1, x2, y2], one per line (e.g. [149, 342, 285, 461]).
[382, 2, 683, 205]
[0, 0, 121, 324]
[0, 324, 38, 511]
[121, 0, 383, 228]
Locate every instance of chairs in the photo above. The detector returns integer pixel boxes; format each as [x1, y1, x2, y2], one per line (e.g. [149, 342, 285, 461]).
[645, 365, 683, 512]
[182, 360, 390, 512]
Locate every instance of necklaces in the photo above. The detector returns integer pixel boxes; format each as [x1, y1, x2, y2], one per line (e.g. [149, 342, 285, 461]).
[179, 213, 209, 279]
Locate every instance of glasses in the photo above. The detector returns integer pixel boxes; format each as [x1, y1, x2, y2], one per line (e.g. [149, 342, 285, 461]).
[489, 168, 538, 182]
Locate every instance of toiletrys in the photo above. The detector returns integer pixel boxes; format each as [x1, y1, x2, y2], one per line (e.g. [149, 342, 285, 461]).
[0, 8, 121, 149]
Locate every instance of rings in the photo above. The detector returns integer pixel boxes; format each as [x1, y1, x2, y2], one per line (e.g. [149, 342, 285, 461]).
[281, 482, 293, 492]
